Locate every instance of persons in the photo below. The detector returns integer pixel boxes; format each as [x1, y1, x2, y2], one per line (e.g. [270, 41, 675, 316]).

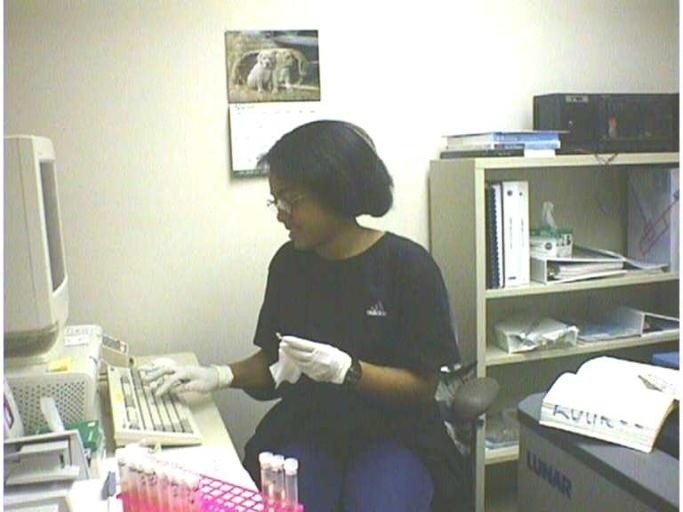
[135, 120, 464, 511]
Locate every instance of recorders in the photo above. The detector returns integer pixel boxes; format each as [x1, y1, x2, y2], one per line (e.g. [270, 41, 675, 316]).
[534, 93, 679, 155]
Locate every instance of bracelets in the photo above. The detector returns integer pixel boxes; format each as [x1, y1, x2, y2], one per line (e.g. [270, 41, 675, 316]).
[342, 353, 363, 388]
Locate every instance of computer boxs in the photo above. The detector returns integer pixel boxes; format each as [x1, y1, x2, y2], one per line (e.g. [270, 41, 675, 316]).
[4, 324, 104, 431]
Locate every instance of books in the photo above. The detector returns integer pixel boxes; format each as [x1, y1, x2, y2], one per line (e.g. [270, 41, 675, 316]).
[484, 410, 520, 458]
[539, 355, 680, 454]
[484, 179, 531, 289]
[627, 165, 680, 273]
[439, 131, 561, 159]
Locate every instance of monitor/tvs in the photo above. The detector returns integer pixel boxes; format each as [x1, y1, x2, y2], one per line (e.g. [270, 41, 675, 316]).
[4, 134, 69, 368]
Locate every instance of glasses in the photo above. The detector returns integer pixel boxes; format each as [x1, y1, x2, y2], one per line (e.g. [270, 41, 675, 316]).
[264, 190, 316, 215]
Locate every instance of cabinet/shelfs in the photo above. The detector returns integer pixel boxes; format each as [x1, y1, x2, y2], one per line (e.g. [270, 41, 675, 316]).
[429, 151, 679, 512]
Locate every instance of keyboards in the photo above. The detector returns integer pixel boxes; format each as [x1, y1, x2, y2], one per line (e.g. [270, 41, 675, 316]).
[108, 365, 204, 446]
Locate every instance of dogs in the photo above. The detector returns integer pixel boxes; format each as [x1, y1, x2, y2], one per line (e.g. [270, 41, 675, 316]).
[247, 49, 296, 96]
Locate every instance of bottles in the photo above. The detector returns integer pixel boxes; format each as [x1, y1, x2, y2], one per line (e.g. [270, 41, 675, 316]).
[115, 443, 299, 512]
